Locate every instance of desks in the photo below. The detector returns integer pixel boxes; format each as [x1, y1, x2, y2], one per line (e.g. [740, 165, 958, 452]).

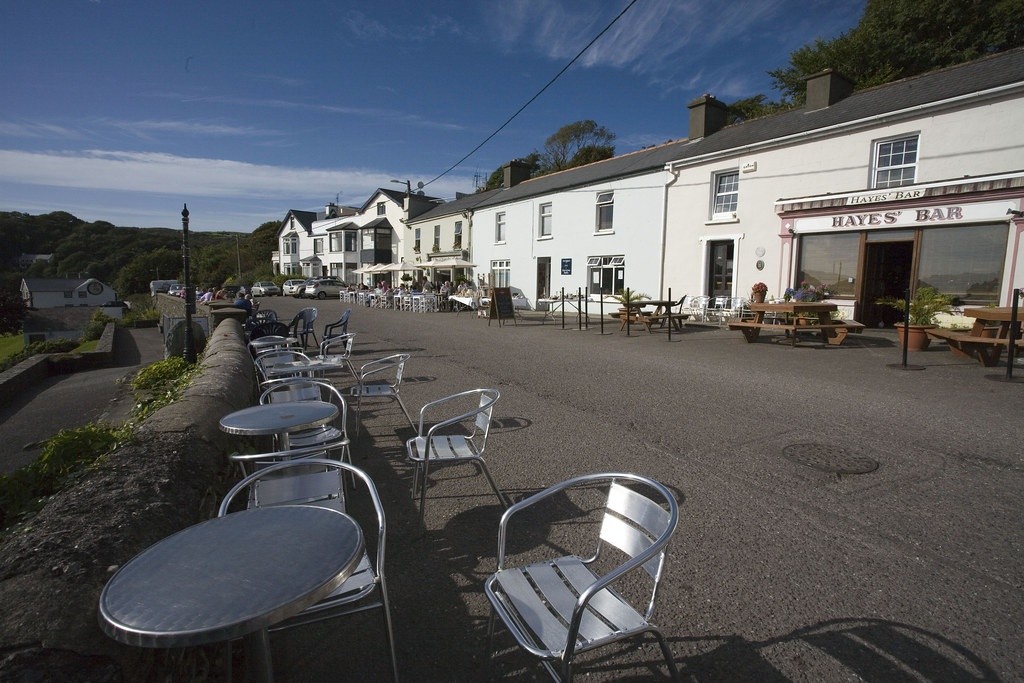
[251, 337, 297, 359]
[537, 297, 593, 326]
[94, 504, 366, 683]
[448, 295, 478, 315]
[220, 399, 340, 475]
[748, 303, 837, 348]
[268, 362, 343, 383]
[619, 301, 677, 335]
[959, 306, 1024, 369]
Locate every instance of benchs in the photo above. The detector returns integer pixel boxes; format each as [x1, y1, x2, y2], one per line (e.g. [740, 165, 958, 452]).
[923, 327, 1024, 368]
[608, 313, 866, 347]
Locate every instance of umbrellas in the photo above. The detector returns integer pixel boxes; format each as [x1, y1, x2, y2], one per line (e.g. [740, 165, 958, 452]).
[351, 258, 478, 282]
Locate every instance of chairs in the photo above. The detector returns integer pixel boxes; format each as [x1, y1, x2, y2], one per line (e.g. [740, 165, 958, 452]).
[406, 387, 508, 537]
[216, 458, 400, 683]
[240, 290, 445, 491]
[480, 470, 681, 683]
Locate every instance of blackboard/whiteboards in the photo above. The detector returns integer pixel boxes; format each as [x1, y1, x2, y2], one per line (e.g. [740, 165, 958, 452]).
[489, 287, 515, 320]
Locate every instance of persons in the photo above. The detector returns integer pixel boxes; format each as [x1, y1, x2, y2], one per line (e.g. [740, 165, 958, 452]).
[346, 276, 469, 313]
[179, 286, 254, 316]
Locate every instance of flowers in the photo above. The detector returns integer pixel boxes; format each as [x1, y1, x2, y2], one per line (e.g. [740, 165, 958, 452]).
[783, 280, 840, 316]
[751, 282, 768, 293]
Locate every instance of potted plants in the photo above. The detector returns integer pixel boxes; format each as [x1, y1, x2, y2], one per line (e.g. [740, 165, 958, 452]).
[604, 288, 652, 313]
[892, 285, 954, 351]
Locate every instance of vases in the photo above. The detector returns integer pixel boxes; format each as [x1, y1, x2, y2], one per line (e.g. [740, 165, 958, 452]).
[798, 316, 810, 326]
[754, 292, 766, 303]
